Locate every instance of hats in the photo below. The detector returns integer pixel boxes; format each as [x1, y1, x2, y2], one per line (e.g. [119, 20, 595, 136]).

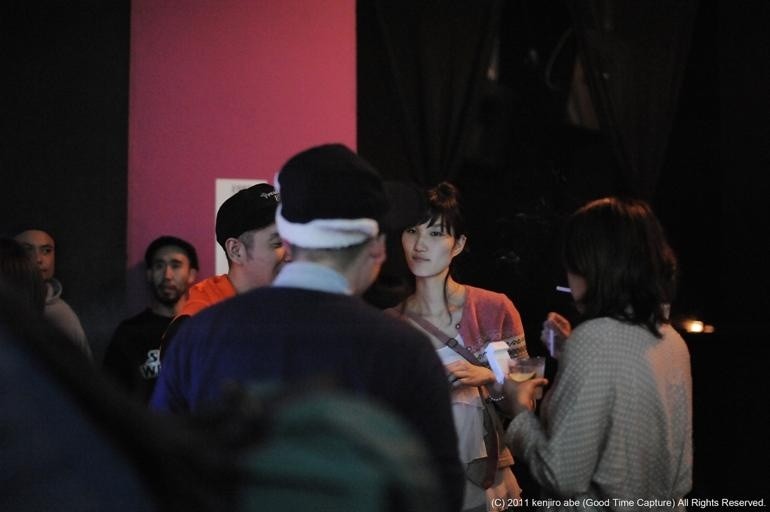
[276, 144, 388, 249]
[146, 236, 199, 272]
[216, 184, 277, 245]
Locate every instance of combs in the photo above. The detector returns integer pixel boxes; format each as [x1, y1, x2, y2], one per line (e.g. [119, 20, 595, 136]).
[554, 284, 571, 292]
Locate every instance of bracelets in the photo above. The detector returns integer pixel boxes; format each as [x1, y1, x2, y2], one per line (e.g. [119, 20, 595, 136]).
[488, 393, 505, 401]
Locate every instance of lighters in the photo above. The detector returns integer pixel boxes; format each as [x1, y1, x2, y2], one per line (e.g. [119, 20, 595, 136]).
[549, 329, 555, 357]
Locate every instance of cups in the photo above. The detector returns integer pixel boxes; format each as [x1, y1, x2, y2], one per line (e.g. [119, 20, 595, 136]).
[508, 358, 547, 401]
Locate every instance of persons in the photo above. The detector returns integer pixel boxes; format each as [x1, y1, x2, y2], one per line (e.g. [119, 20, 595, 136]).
[3, 139, 469, 511]
[493, 195, 698, 512]
[374, 181, 536, 512]
